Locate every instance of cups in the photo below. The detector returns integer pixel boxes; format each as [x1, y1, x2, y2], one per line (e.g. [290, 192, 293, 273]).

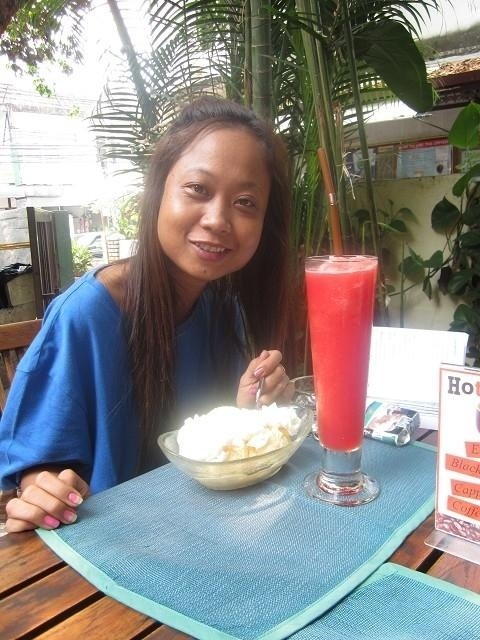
[305, 253, 381, 507]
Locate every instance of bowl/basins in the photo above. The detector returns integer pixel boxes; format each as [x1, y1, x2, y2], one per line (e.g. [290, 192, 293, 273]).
[289, 375, 317, 433]
[157, 407, 312, 491]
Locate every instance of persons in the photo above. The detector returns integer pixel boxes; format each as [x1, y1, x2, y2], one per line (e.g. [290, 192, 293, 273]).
[0, 105, 295, 533]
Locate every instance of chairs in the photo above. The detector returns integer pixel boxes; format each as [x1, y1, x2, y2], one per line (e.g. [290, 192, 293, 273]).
[0, 319, 54, 413]
